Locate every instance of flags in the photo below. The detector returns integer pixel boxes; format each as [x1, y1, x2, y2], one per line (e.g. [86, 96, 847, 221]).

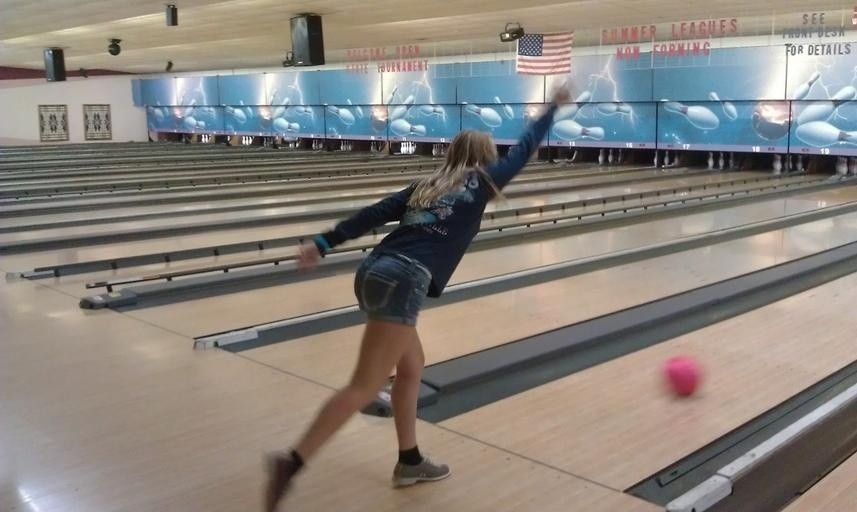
[516, 31, 573, 74]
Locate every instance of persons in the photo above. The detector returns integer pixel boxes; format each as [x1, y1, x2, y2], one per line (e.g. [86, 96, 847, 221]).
[267, 81, 571, 512]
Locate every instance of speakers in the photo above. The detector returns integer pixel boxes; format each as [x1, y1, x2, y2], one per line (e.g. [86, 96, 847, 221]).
[43, 48, 66, 82]
[287, 17, 325, 66]
[166, 7, 178, 26]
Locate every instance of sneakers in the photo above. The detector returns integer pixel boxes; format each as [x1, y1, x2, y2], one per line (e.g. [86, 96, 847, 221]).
[391, 455, 451, 486]
[260, 448, 301, 512]
